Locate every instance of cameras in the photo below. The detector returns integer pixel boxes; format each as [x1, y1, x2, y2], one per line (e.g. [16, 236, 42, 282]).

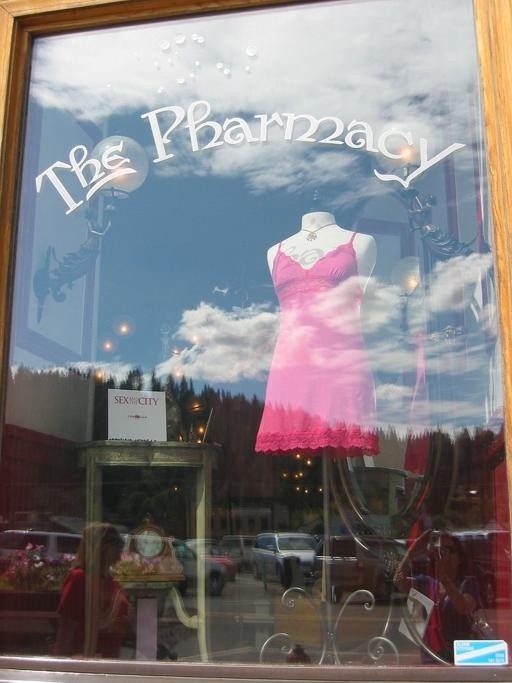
[428, 531, 442, 548]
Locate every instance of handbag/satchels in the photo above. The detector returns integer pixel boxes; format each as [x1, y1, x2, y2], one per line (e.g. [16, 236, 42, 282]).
[458, 575, 497, 640]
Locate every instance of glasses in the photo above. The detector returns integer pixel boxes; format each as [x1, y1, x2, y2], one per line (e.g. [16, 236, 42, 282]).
[104, 537, 127, 545]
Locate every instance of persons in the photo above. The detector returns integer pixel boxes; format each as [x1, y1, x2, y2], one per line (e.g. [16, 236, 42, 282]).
[391, 529, 481, 663]
[255, 211, 378, 458]
[53, 522, 130, 657]
